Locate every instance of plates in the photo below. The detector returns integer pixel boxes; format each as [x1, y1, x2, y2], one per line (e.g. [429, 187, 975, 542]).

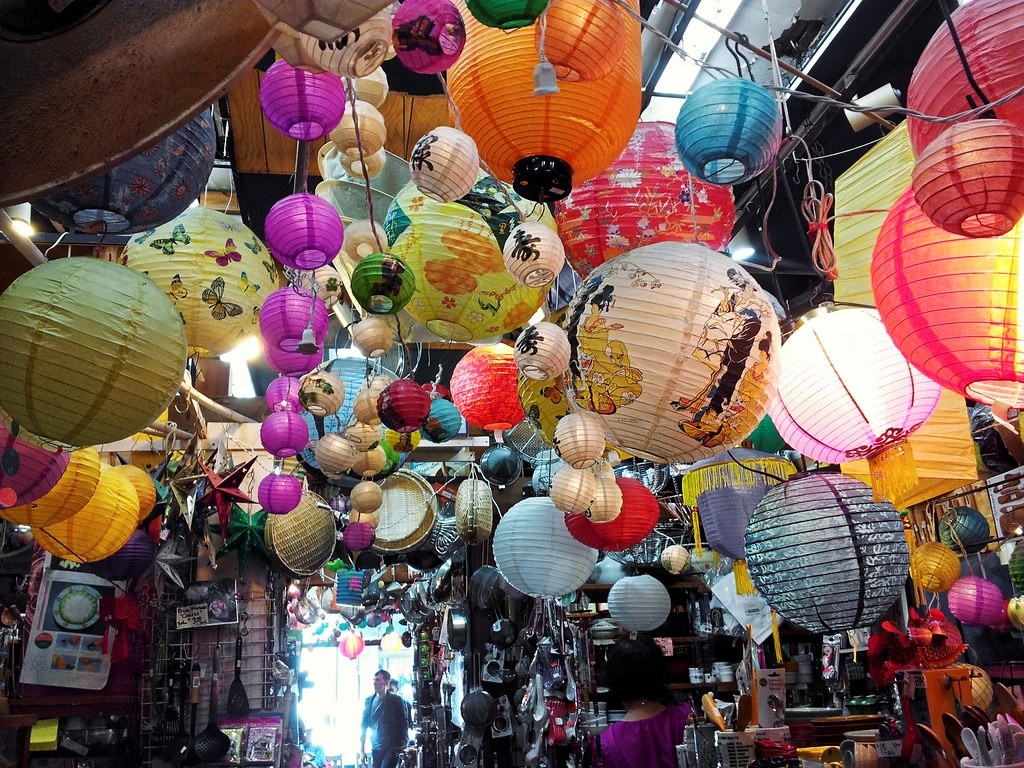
[843, 730, 880, 741]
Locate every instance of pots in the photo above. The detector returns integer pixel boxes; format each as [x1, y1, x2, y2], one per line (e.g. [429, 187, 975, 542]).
[489, 587, 519, 650]
[63, 714, 130, 747]
[459, 741, 477, 768]
[447, 603, 467, 649]
[480, 429, 522, 490]
[461, 651, 497, 730]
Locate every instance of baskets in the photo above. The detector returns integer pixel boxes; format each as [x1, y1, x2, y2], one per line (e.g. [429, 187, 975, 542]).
[264, 490, 336, 579]
[369, 467, 438, 555]
[455, 479, 493, 548]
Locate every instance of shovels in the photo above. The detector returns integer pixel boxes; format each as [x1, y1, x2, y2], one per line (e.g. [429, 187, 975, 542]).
[163, 668, 192, 764]
[227, 635, 249, 715]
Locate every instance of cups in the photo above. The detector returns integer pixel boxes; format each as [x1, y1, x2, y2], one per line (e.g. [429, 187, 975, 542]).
[713, 725, 791, 768]
[785, 672, 798, 684]
[962, 759, 1024, 768]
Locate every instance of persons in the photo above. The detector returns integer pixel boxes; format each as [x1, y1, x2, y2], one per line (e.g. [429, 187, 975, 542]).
[359, 670, 413, 768]
[589, 634, 700, 768]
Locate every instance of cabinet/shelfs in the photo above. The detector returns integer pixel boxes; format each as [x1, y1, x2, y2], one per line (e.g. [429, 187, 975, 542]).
[569, 574, 739, 702]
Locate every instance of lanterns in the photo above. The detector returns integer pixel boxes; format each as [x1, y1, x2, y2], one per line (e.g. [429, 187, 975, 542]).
[1, 0, 1024, 630]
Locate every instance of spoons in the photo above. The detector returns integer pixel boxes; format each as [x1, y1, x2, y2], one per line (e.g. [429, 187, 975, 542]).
[916, 705, 1024, 768]
[879, 717, 906, 768]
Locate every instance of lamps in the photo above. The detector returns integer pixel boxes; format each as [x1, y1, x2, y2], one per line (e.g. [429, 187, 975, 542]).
[842, 83, 902, 133]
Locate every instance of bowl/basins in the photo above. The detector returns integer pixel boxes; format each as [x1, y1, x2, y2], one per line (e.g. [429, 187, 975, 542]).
[689, 662, 734, 684]
[579, 701, 609, 735]
[820, 739, 878, 768]
[588, 621, 619, 639]
